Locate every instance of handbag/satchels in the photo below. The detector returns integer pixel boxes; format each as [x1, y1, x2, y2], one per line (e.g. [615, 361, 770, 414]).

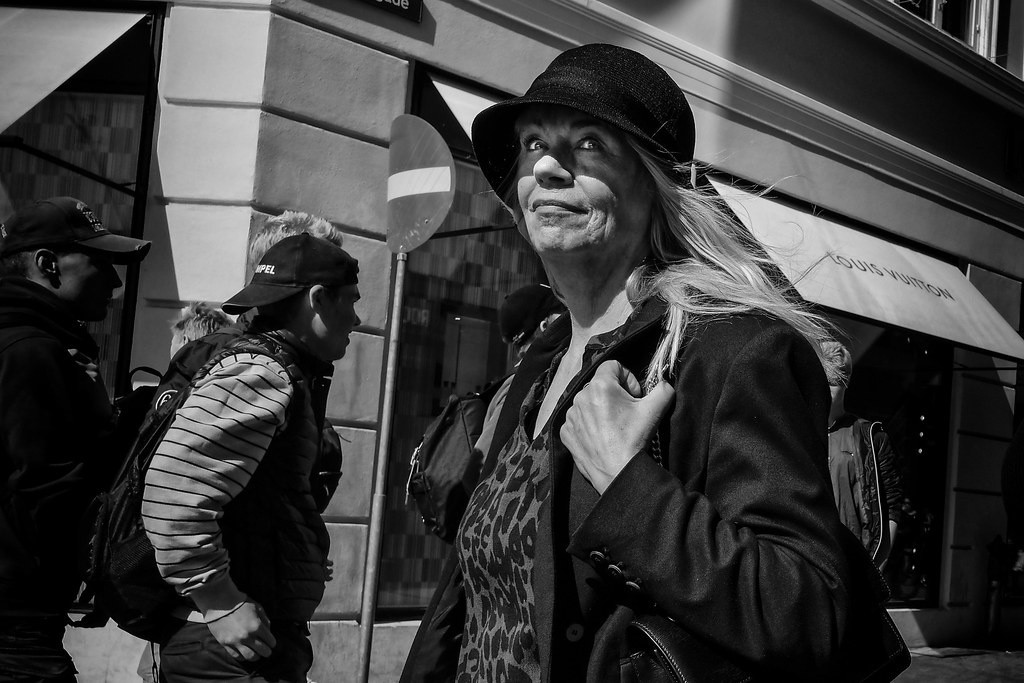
[620, 550, 912, 683]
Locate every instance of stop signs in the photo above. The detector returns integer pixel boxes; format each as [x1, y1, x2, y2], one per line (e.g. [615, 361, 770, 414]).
[389, 113, 457, 260]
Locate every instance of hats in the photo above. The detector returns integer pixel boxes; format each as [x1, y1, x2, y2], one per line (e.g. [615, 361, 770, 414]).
[471, 41, 696, 209]
[220, 232, 360, 315]
[499, 284, 568, 351]
[0, 196, 152, 265]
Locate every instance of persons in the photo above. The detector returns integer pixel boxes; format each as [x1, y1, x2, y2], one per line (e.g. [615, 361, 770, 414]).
[396, 43, 915, 683]
[0, 196, 363, 683]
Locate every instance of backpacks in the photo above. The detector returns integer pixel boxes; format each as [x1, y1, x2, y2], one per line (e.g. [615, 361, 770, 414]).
[87, 346, 299, 646]
[405, 373, 517, 544]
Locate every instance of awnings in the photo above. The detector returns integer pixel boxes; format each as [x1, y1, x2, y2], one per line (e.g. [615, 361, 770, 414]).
[423, 70, 1024, 361]
[0, 7, 153, 136]
